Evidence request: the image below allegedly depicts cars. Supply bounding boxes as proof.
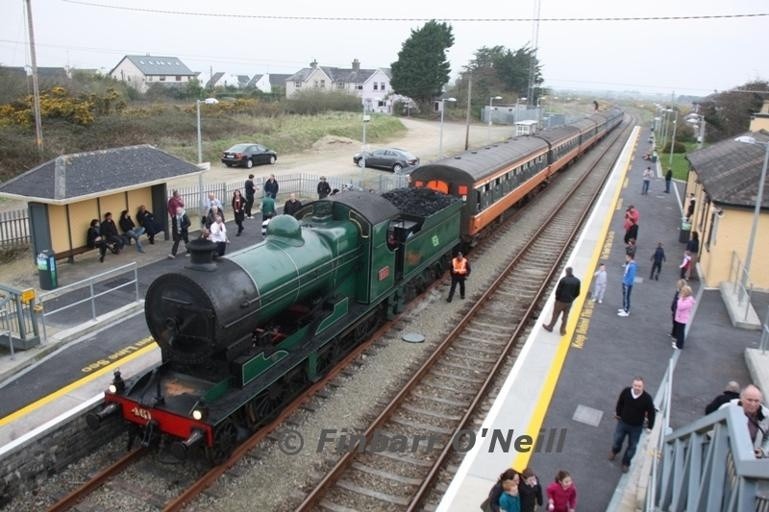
[353,146,420,173]
[221,143,277,168]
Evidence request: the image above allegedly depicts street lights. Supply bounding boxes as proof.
[537,95,583,127]
[516,97,528,120]
[196,97,220,215]
[489,95,502,143]
[652,100,679,166]
[690,113,706,151]
[362,96,383,144]
[734,135,769,305]
[439,97,457,156]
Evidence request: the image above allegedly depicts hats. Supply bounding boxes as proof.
[320,176,326,180]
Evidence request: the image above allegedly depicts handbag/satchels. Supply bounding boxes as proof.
[481,496,496,512]
[112,244,120,254]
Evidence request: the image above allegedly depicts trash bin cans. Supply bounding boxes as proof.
[679,223,691,242]
[36,250,58,290]
[652,156,657,163]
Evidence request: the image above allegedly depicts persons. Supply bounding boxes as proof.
[617,252,638,318]
[447,251,471,300]
[546,470,577,512]
[720,384,768,458]
[87,219,118,263]
[168,173,340,260]
[642,167,654,194]
[672,285,696,350]
[101,212,128,254]
[499,478,521,511]
[137,205,161,244]
[621,192,701,283]
[610,375,655,472]
[592,263,608,303]
[667,279,687,338]
[663,167,672,193]
[542,267,580,335]
[119,210,146,253]
[704,381,740,439]
[521,467,543,511]
[487,469,522,511]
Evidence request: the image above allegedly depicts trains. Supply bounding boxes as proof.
[92,105,625,475]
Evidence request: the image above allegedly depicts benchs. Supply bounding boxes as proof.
[54,234,131,264]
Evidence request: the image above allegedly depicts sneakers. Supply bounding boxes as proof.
[617,309,628,316]
[608,450,615,460]
[543,323,552,332]
[622,465,628,472]
